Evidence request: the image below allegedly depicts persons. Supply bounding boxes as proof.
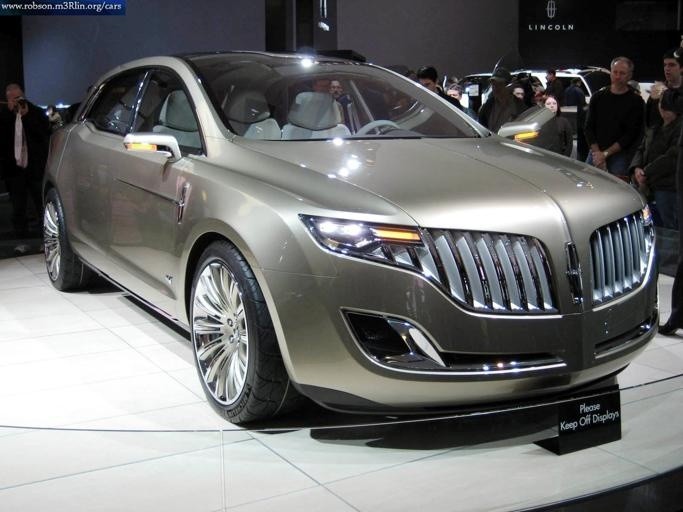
[655,245,681,336]
[581,57,645,176]
[46,104,61,126]
[310,54,587,147]
[625,93,681,230]
[62,86,91,124]
[0,83,54,254]
[644,52,681,132]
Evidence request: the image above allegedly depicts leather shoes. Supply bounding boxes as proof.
[658,322,683,337]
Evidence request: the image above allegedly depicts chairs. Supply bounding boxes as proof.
[224,85,281,140]
[153,91,202,150]
[281,91,352,140]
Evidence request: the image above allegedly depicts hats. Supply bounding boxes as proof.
[488,67,513,82]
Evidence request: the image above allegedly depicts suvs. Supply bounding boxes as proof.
[40,50,666,424]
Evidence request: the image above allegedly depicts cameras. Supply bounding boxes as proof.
[13,96,25,108]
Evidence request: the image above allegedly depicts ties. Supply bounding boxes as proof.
[13,113,24,162]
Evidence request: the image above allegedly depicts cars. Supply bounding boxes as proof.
[39,101,76,125]
[436,65,671,164]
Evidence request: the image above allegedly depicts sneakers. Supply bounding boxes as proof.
[14,243,45,254]
